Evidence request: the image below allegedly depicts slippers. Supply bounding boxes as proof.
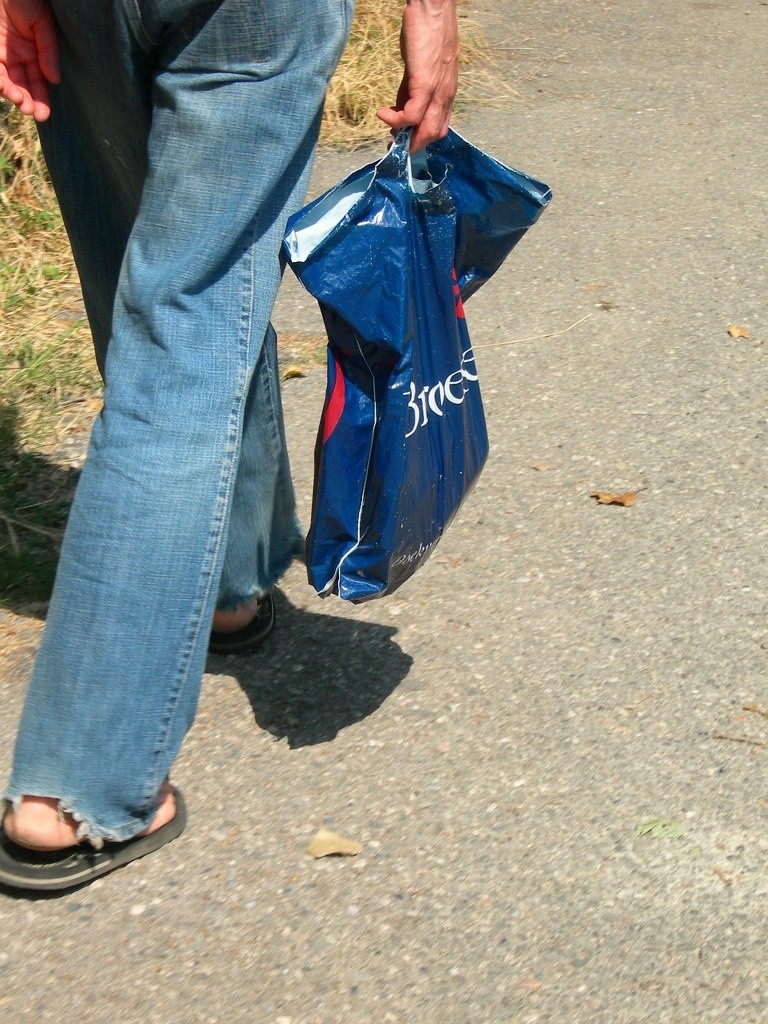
[206,589,272,654]
[0,784,186,890]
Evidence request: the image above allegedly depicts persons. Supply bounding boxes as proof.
[0,0,459,890]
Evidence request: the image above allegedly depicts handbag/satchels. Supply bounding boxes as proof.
[284,124,552,603]
